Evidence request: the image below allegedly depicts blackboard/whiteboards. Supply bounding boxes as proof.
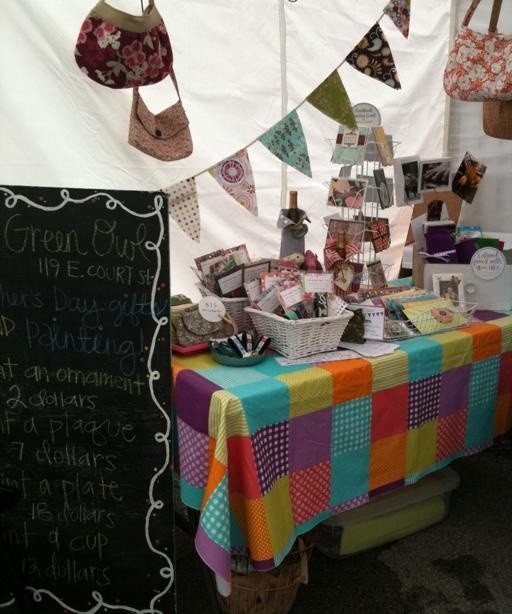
[0,184,179,614]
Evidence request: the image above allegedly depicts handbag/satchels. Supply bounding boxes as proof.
[171,303,222,347]
[75,0,193,86]
[128,86,193,161]
[443,0,512,139]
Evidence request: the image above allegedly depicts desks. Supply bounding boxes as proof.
[161,302,511,614]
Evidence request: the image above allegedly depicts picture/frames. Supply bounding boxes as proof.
[432,272,465,310]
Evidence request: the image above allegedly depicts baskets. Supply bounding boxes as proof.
[196,281,355,359]
[216,533,318,614]
[383,299,479,341]
